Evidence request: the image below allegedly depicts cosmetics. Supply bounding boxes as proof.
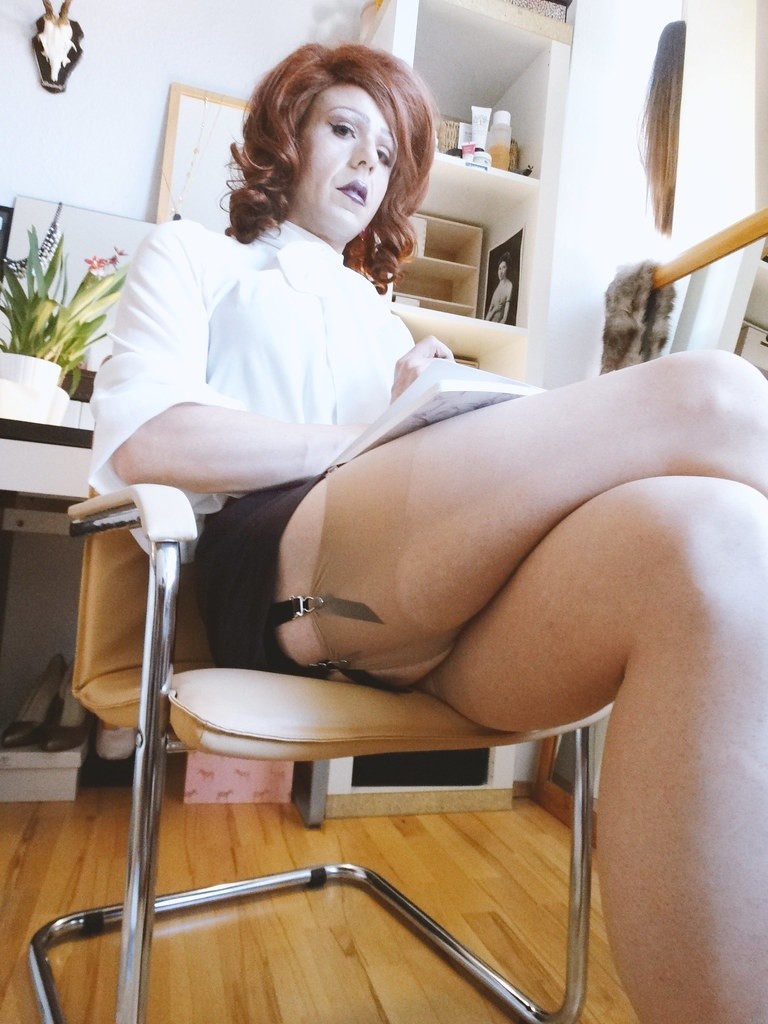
[446,104,512,169]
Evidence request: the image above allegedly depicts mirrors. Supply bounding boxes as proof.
[155,82,251,240]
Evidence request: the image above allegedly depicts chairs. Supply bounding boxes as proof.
[22,485,617,1024]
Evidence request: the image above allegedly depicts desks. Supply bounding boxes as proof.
[0,416,95,803]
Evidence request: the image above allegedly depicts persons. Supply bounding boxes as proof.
[484,254,511,324]
[88,46,768,1024]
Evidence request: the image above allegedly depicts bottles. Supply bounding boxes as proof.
[488,110,512,169]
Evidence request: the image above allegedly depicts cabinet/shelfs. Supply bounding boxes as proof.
[321,0,577,826]
[393,212,483,316]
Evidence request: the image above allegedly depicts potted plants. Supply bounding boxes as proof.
[0,225,131,423]
